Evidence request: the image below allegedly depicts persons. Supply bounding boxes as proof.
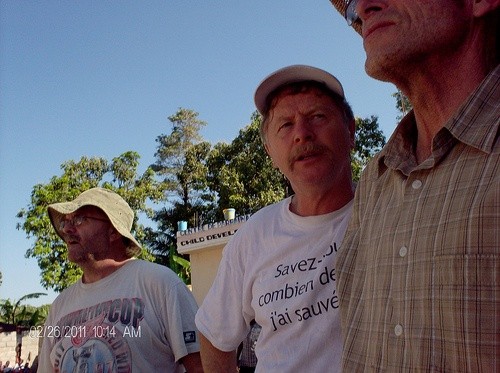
[0,355,38,373]
[329,0,500,372]
[195,64,359,373]
[35,188,205,373]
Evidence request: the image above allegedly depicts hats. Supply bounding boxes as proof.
[46,187,143,258]
[253,65,346,115]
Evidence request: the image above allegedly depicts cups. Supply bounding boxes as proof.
[177,221,187,230]
[222,208,235,220]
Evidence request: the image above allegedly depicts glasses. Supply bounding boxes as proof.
[345,0,363,26]
[58,214,110,231]
